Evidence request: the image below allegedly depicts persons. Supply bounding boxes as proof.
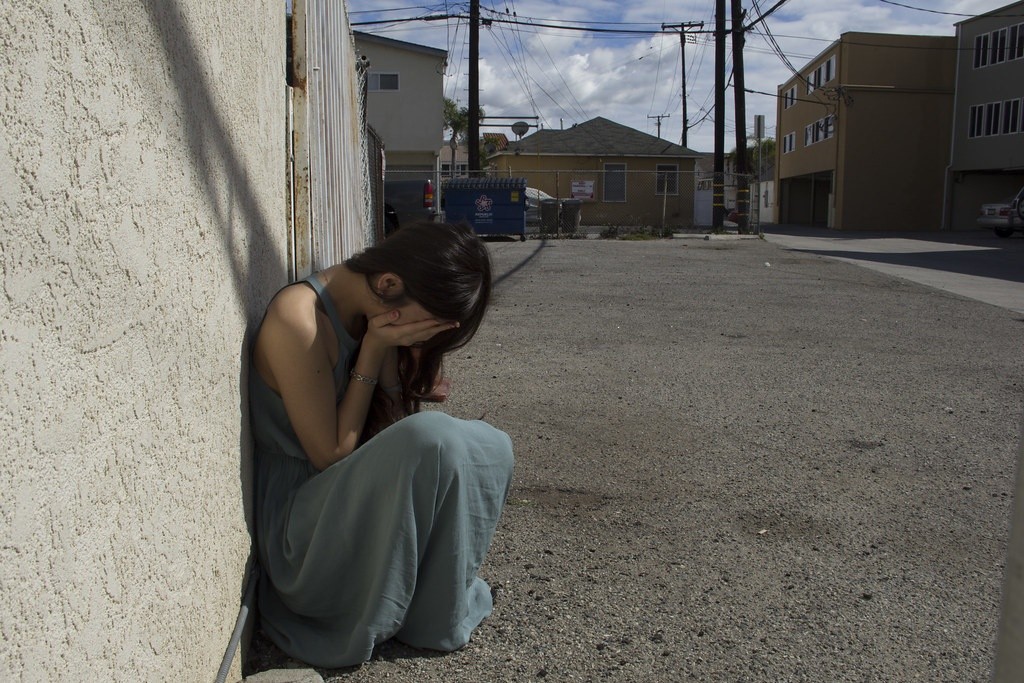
[247,220,514,669]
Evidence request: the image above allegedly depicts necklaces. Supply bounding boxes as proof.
[320,270,329,290]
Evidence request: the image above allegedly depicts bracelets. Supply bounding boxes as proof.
[350,367,377,386]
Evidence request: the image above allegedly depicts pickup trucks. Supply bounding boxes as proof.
[976,184,1024,239]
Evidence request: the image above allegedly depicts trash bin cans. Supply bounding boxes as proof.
[539,198,560,235]
[561,197,584,234]
[440,176,530,242]
